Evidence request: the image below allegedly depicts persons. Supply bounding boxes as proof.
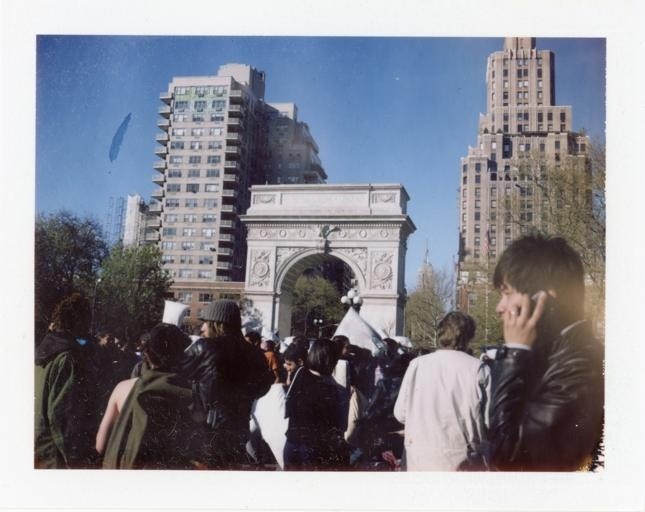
[35,292,496,471]
[473,231,606,471]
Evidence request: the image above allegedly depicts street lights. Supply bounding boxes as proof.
[478,277,489,344]
[89,277,103,337]
[343,288,363,314]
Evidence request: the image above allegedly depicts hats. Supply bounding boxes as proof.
[197,299,241,325]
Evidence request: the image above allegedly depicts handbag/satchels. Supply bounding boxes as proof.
[284,396,291,418]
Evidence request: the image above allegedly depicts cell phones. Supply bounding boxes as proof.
[529,292,558,329]
